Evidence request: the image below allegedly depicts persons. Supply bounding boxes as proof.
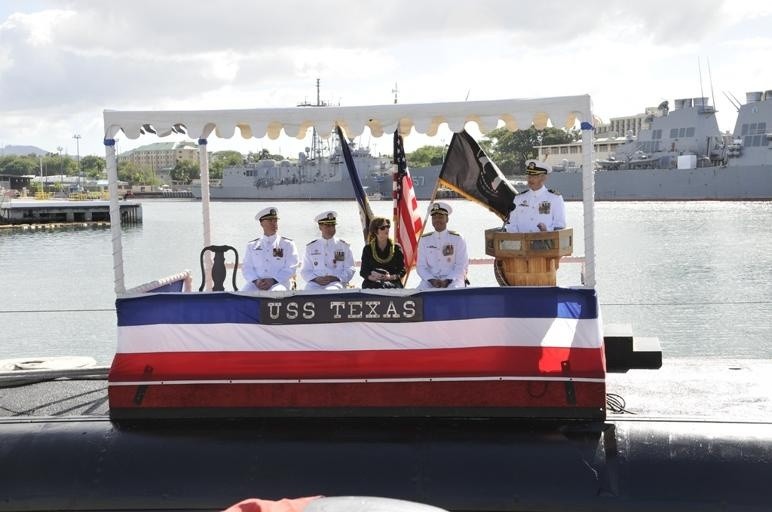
[300,210,356,290]
[359,218,406,288]
[504,158,566,232]
[234,206,302,293]
[415,201,468,288]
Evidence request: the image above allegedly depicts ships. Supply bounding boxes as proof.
[189,78,442,199]
[507,90,772,200]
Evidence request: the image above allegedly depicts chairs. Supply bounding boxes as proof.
[198,243,240,292]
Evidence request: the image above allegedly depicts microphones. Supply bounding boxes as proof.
[503,202,517,220]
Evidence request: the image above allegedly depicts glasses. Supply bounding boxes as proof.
[377,224,390,230]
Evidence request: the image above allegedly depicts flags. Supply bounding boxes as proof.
[441,127,519,223]
[335,126,375,243]
[392,126,422,268]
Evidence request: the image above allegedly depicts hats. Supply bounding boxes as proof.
[524,159,552,175]
[427,201,452,216]
[255,206,278,220]
[314,211,338,224]
